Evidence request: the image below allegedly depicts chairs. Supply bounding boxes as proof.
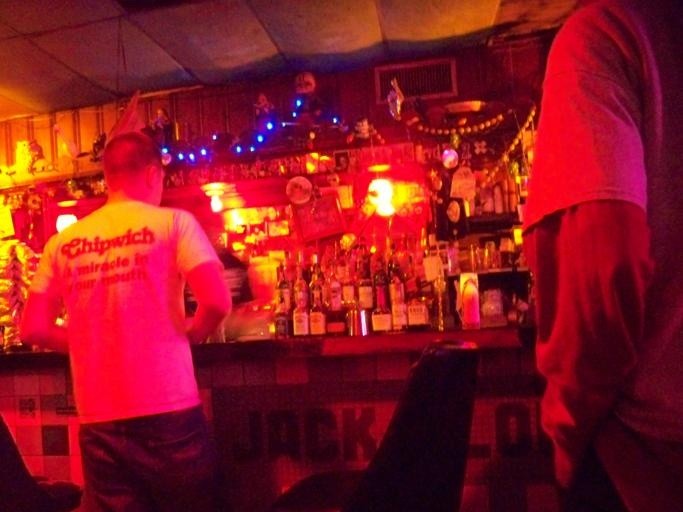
[273,344,480,512]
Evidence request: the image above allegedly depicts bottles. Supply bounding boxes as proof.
[59,136,78,176]
[276,145,525,337]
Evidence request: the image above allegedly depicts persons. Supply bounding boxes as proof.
[14,130,232,512]
[519,0,680,510]
[159,198,274,344]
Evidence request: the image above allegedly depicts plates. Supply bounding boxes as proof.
[184,267,245,305]
